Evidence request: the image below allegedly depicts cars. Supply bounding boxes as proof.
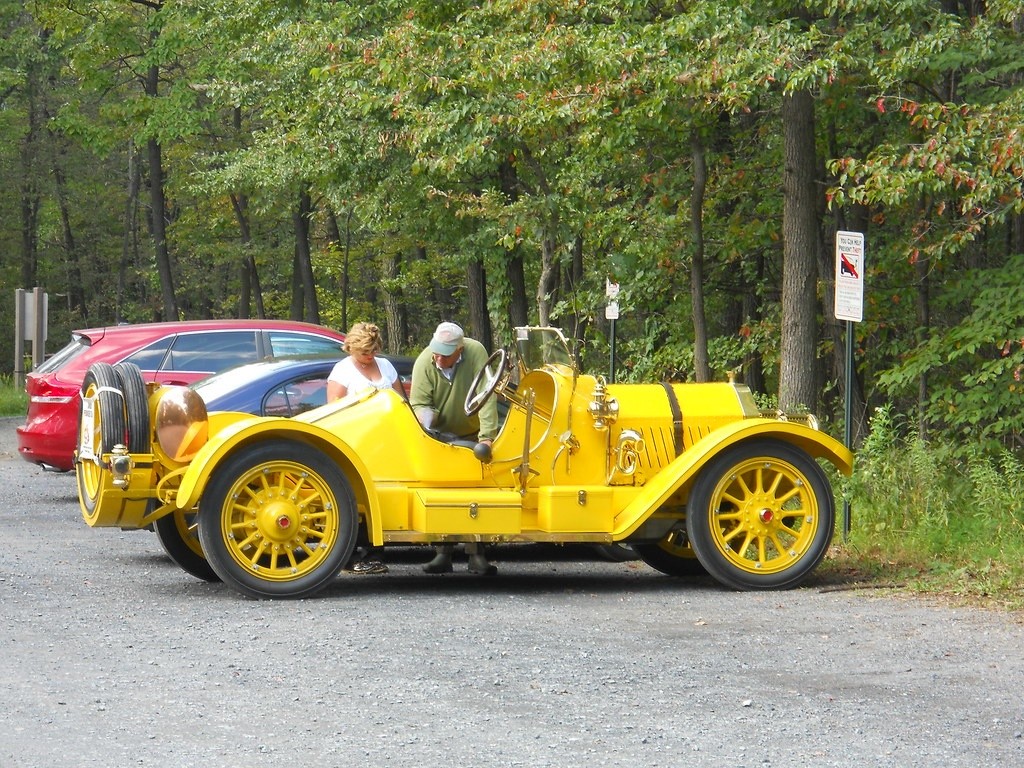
[70,322,857,602]
[119,351,520,453]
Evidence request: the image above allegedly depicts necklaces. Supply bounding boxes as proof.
[362,364,367,368]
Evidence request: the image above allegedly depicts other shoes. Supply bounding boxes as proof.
[468,554,497,575]
[421,554,453,573]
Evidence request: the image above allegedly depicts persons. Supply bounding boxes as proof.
[327,322,407,572]
[410,321,498,574]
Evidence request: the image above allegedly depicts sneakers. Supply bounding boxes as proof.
[347,562,372,573]
[367,561,389,572]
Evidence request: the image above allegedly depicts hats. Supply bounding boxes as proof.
[429,322,464,356]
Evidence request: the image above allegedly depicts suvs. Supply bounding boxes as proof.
[14,317,413,476]
